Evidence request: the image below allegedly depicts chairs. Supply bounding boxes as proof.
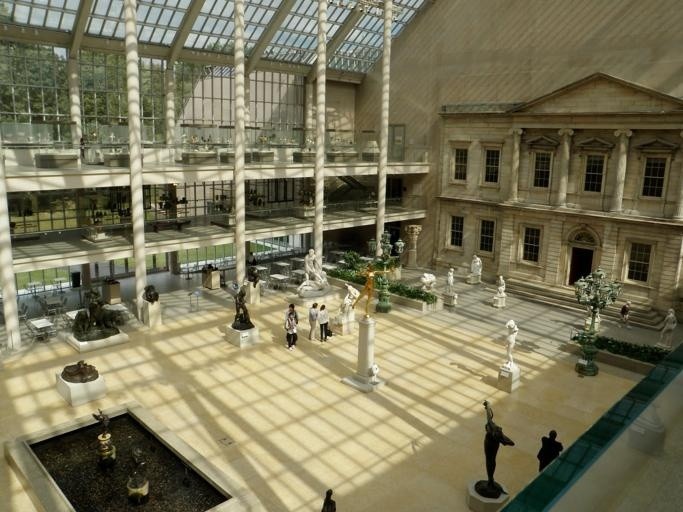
[17,290,68,339]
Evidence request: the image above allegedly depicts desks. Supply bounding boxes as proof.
[252,254,338,287]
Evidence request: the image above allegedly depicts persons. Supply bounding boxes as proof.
[615,301,633,329]
[284,303,299,351]
[658,307,677,346]
[352,263,395,316]
[322,489,336,512]
[505,319,519,367]
[234,287,251,323]
[537,429,563,473]
[336,283,360,319]
[297,249,329,296]
[482,398,516,487]
[447,254,508,298]
[308,303,332,342]
